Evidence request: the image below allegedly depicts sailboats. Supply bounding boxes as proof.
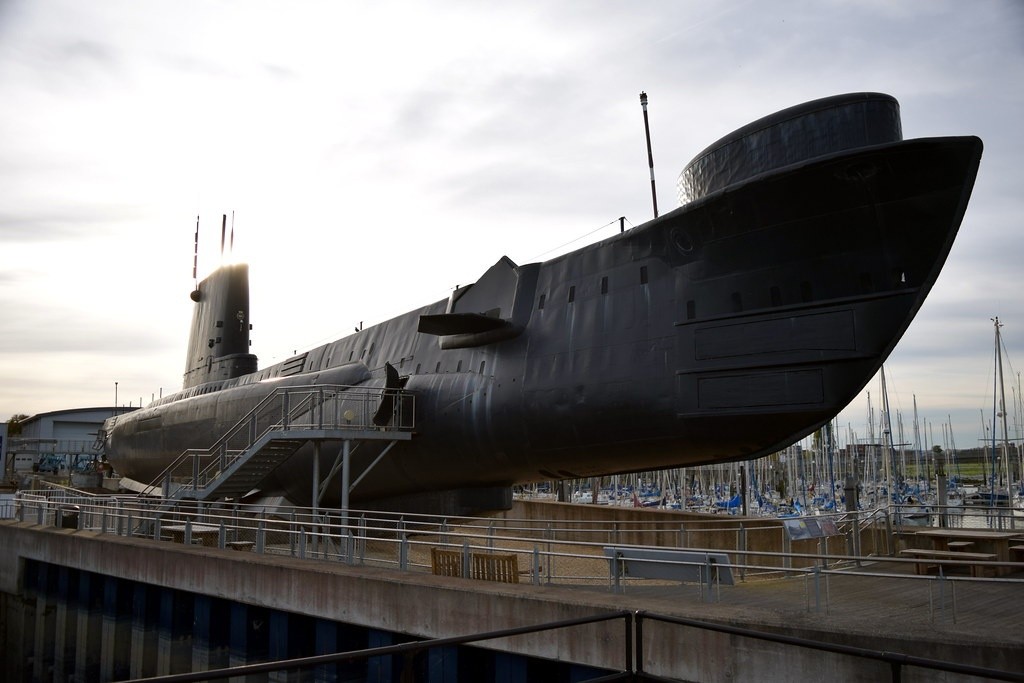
[511,315,1024,531]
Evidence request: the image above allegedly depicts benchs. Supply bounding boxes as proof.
[431,546,542,583]
[900,539,1024,579]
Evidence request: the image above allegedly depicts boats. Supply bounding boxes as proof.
[92,88,986,540]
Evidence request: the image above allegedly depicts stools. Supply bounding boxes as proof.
[229,541,256,551]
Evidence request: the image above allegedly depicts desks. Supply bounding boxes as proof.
[914,529,1024,578]
[161,525,235,547]
